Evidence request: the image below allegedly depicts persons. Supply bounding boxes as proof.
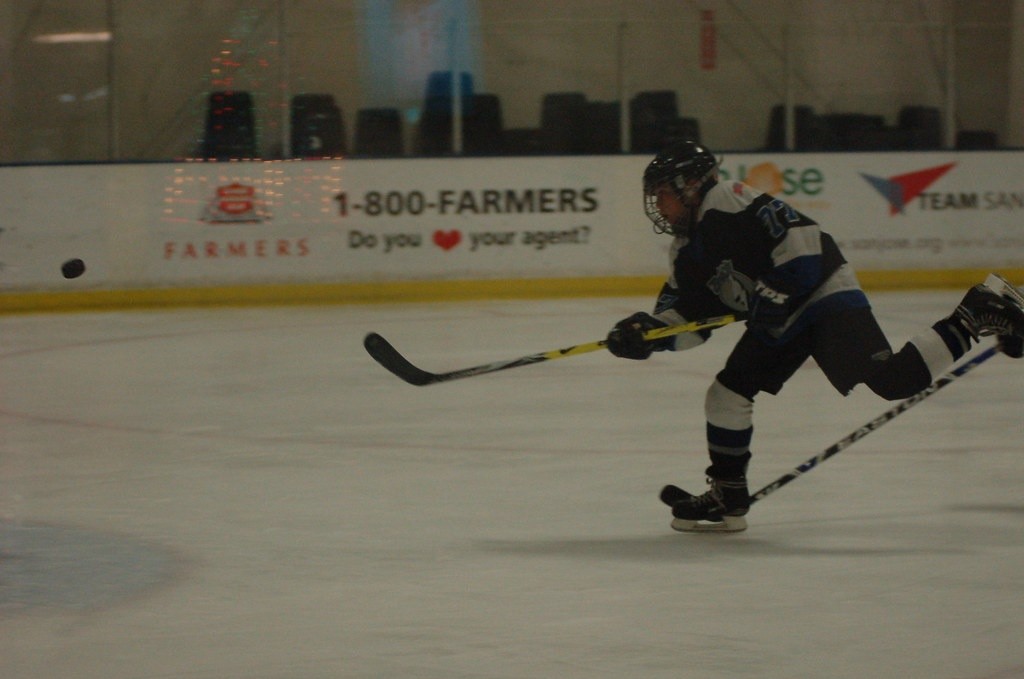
[606,140,1024,521]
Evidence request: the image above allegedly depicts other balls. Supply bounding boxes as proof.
[61,258,85,278]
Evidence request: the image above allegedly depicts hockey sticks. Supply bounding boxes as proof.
[362,305,752,389]
[656,344,1003,513]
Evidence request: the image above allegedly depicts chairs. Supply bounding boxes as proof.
[204,71,705,157]
[766,103,999,151]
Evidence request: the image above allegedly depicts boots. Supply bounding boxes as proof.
[671,451,752,533]
[949,272,1024,358]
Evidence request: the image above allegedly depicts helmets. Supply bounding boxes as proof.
[643,139,724,238]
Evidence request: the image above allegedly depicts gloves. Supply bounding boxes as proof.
[748,282,790,332]
[607,312,676,360]
